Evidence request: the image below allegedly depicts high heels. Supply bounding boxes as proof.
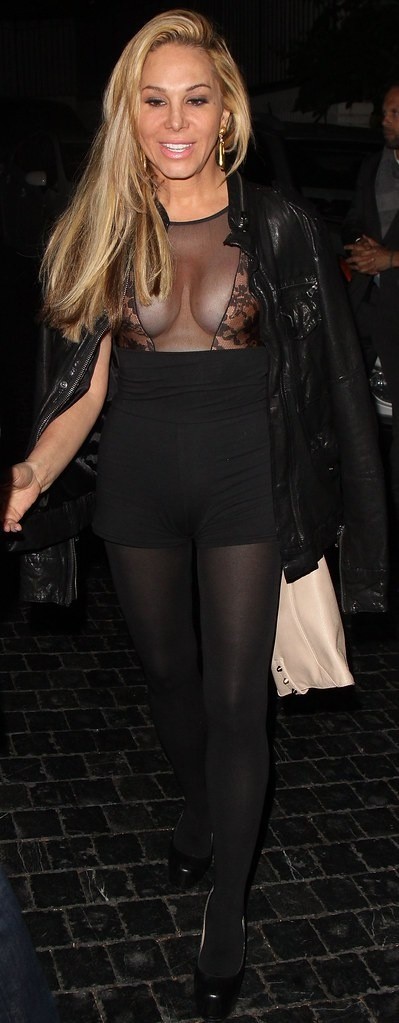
[194,884,247,1023]
[167,807,214,891]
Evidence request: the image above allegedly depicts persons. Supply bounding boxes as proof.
[3,8,339,1022]
[337,83,399,641]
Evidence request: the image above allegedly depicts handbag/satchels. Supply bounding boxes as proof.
[271,548,357,697]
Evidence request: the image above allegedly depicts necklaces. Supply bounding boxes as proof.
[394,149,399,164]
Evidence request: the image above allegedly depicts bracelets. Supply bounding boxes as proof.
[387,250,395,267]
